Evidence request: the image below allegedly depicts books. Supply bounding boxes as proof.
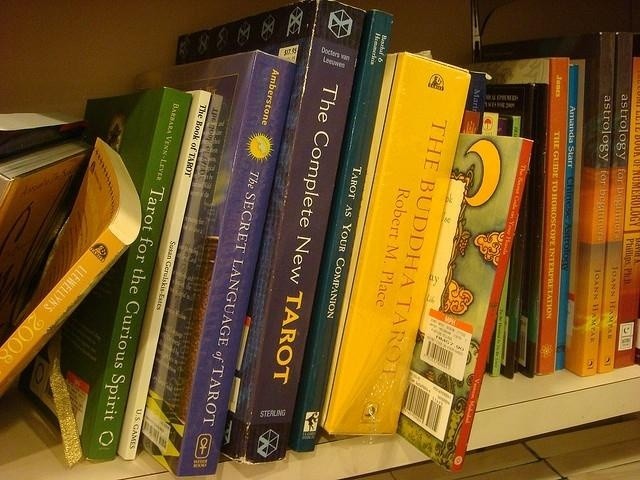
[0,87,211,470]
[135,1,640,479]
